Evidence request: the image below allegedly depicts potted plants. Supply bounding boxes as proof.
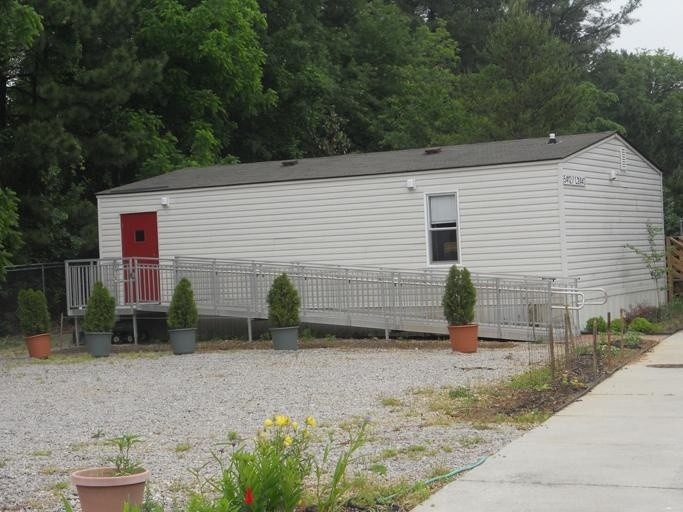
[265,271,302,350]
[441,264,478,353]
[71,433,149,512]
[166,277,198,354]
[15,288,51,358]
[81,281,117,357]
